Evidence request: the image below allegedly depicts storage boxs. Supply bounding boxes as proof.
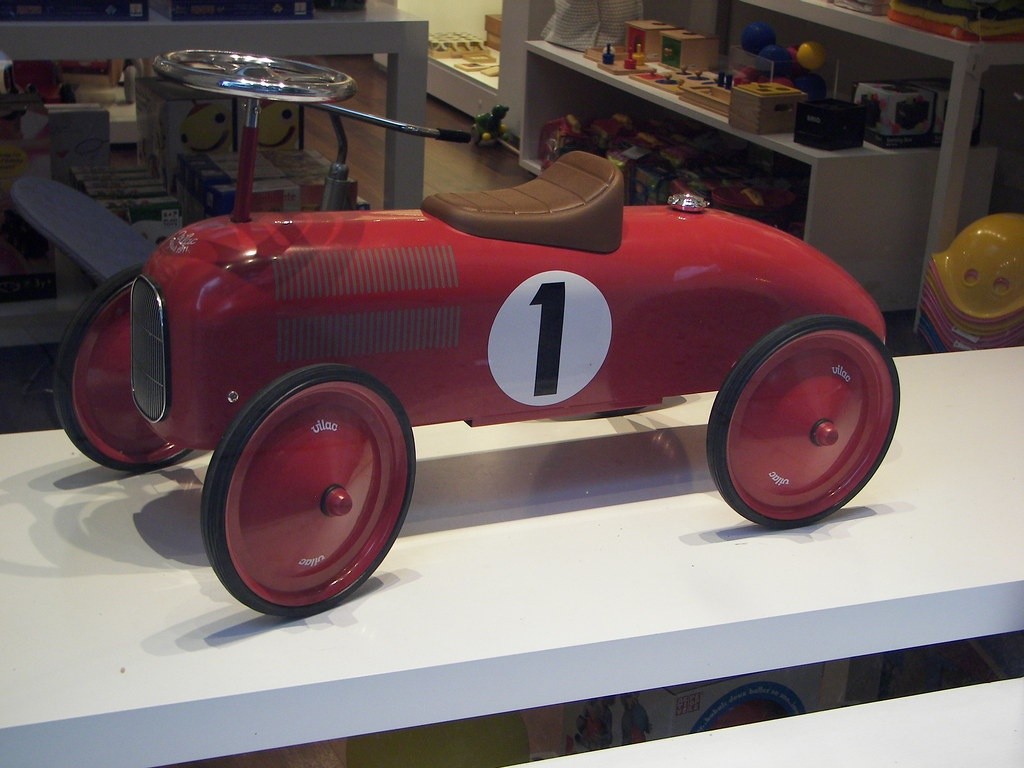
[728,82,808,135]
[794,97,866,151]
[561,661,824,755]
[906,78,984,148]
[854,81,938,147]
[624,19,677,63]
[658,28,721,73]
[485,14,502,51]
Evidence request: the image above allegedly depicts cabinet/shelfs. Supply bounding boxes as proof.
[516,0,1024,319]
[0,0,433,388]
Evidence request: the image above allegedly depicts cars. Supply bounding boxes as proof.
[53,48,903,624]
